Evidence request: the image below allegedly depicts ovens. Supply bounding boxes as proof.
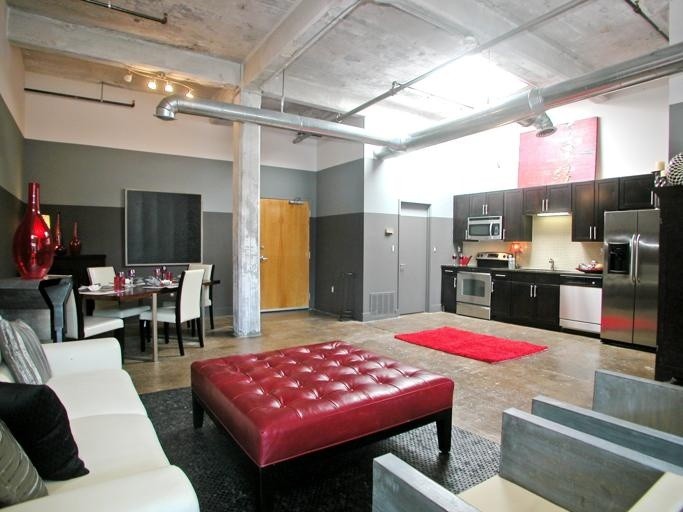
[455,272,491,307]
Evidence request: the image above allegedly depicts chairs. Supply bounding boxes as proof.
[532,365,683,469]
[0,254,222,359]
[369,405,683,512]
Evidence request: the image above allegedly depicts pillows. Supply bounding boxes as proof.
[0,316,54,386]
[0,420,48,505]
[1,382,88,483]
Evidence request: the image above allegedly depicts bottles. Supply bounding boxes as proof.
[52,212,66,255]
[453,254,457,265]
[458,253,463,265]
[68,221,81,255]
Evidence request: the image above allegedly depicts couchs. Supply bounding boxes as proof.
[0,312,200,512]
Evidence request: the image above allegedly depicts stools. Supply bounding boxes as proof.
[191,339,454,509]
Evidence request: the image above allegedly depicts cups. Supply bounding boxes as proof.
[154,265,173,282]
[113,271,126,292]
[128,269,136,277]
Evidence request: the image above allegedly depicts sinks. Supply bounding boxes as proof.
[523,268,566,273]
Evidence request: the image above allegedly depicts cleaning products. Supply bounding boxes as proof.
[508,255,515,270]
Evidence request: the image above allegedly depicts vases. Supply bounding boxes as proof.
[69,221,82,255]
[48,209,66,254]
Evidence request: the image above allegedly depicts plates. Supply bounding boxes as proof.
[576,266,602,273]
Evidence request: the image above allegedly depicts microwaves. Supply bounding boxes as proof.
[467,217,502,240]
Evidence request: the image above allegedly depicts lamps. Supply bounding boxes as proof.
[121,68,193,100]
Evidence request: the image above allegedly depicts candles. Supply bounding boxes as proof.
[655,159,665,170]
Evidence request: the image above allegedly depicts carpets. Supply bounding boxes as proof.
[394,324,551,366]
[136,385,501,511]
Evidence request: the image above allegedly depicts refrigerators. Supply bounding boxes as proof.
[599,209,659,348]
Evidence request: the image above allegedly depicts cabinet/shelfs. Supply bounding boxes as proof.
[513,271,559,330]
[572,177,618,244]
[438,263,455,311]
[490,270,514,323]
[501,188,533,242]
[452,194,470,234]
[619,173,660,211]
[523,185,572,217]
[652,184,682,390]
[471,189,502,218]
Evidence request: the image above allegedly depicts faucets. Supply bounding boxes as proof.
[548,258,555,270]
